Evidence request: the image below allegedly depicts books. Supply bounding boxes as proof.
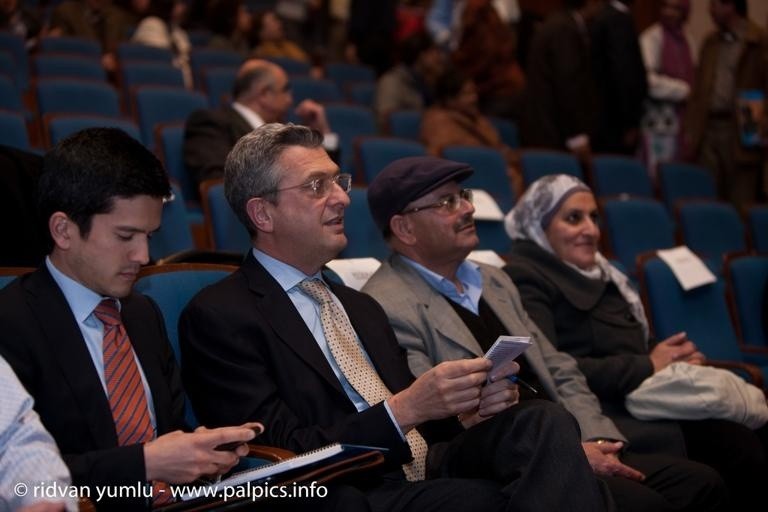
[181,440,344,504]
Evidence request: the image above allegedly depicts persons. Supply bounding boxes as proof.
[501,172,767,510]
[177,122,611,511]
[359,156,730,512]
[0,0,767,267]
[1,356,79,511]
[1,126,371,511]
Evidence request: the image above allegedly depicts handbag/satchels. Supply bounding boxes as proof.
[625,364,767,433]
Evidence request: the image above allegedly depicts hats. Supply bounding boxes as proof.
[367,153,473,232]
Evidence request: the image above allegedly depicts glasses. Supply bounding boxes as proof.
[260,170,357,200]
[406,186,477,214]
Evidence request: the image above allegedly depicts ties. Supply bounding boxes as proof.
[92,298,180,505]
[299,275,430,486]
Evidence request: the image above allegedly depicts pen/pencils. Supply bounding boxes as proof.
[507,375,537,394]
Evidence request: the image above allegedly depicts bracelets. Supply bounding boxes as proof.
[594,438,607,445]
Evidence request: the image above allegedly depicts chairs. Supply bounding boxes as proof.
[0,34,768,512]
